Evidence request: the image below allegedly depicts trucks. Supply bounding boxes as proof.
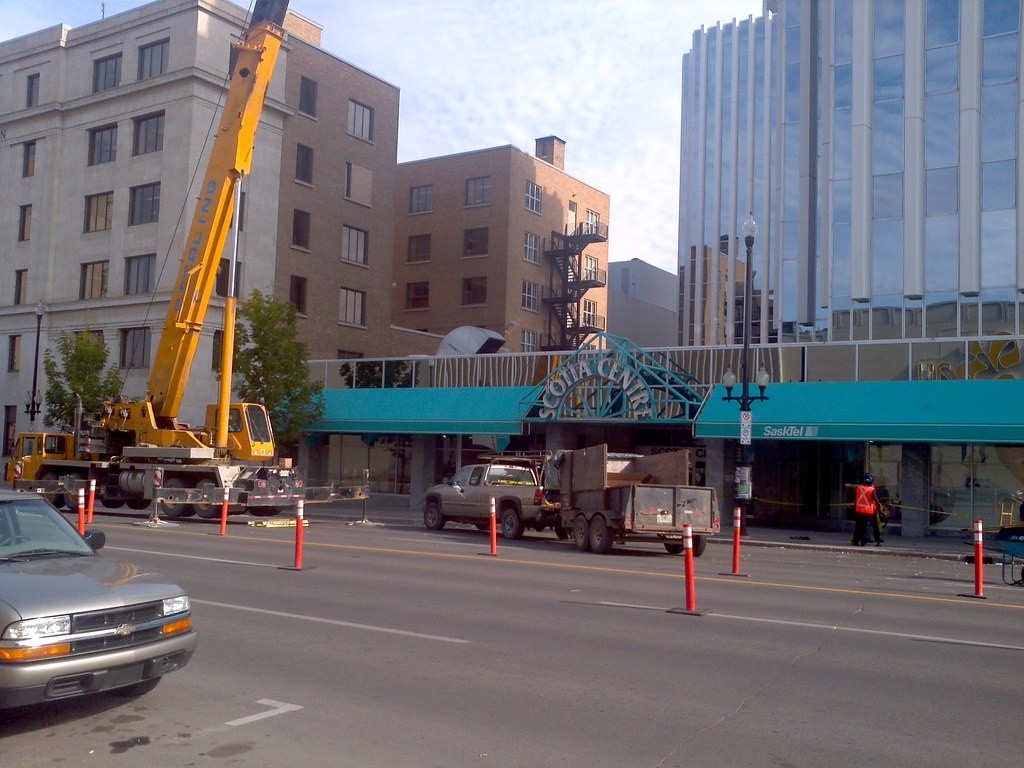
[546,443,720,556]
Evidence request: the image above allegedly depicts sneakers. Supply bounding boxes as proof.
[851,540,860,546]
[876,544,882,547]
[866,539,874,542]
[875,538,884,542]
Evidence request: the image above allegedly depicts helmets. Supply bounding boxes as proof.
[862,472,874,483]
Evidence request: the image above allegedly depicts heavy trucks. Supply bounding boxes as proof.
[5,430,305,517]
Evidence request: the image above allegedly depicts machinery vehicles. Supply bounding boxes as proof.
[76,0,296,473]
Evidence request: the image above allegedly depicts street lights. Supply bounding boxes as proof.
[721,209,771,537]
[22,299,42,454]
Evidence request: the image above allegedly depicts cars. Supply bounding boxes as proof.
[1,487,198,716]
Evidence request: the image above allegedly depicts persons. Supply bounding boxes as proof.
[848,472,885,548]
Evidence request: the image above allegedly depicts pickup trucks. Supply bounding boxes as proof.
[423,449,573,541]
